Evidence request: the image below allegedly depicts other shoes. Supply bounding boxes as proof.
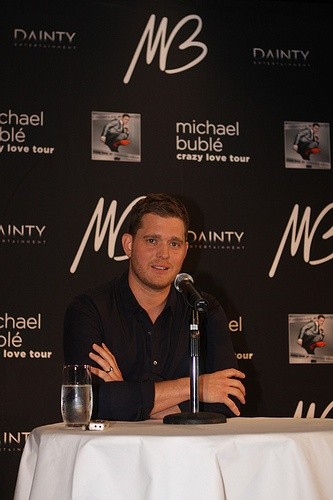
[113,139,130,148]
[309,341,326,350]
[305,148,320,156]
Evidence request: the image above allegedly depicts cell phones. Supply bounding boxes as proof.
[89,419,109,431]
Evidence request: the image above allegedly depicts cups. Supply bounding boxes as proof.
[58,365,94,430]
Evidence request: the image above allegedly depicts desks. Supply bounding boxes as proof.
[12,417,332,499]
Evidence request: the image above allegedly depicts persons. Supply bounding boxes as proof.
[297,315,327,363]
[292,123,321,168]
[100,114,130,161]
[62,192,247,422]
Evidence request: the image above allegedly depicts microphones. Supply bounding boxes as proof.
[174,273,209,313]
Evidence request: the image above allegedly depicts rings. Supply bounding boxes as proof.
[104,367,113,373]
